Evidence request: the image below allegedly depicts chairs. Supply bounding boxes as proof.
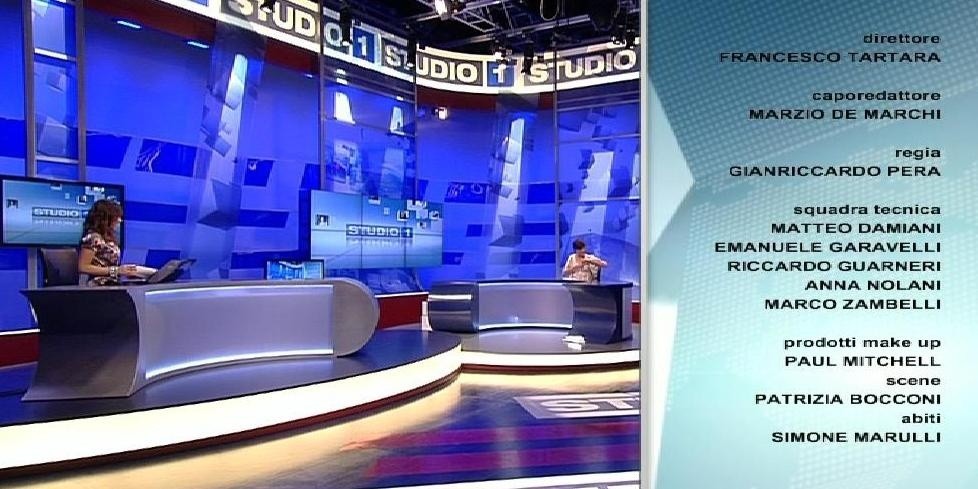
[42,248,79,285]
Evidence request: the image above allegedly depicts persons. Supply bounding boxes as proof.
[76,200,137,286]
[562,240,607,283]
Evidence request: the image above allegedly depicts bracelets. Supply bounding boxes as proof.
[109,265,119,278]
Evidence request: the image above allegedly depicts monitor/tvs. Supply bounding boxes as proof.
[264,259,324,280]
[0,175,125,247]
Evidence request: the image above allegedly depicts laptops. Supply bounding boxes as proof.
[122,260,182,284]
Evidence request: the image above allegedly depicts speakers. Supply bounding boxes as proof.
[585,1,621,32]
